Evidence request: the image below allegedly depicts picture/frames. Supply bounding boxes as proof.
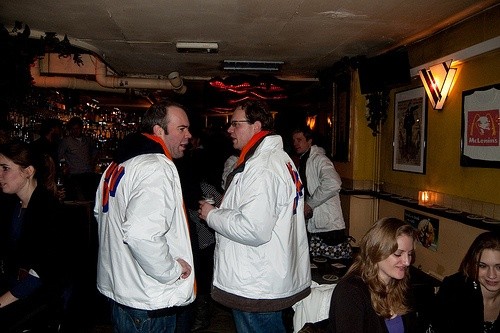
[331,69,353,162]
[459,83,500,170]
[391,86,428,175]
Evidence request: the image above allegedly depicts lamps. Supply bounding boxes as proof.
[418,59,457,110]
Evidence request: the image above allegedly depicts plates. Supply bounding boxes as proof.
[466,215,485,219]
[313,258,327,263]
[483,219,500,223]
[323,274,338,282]
[391,196,403,198]
[383,194,392,195]
[446,211,462,213]
[432,207,448,209]
[408,200,418,203]
[399,198,411,200]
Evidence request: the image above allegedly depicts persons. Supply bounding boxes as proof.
[0,139,82,333]
[58,117,92,188]
[291,125,346,246]
[198,99,312,333]
[34,154,66,199]
[93,100,197,333]
[432,232,500,333]
[329,217,432,333]
[191,132,203,149]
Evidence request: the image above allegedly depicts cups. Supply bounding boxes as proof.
[202,194,216,207]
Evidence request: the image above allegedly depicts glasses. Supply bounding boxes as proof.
[231,120,248,128]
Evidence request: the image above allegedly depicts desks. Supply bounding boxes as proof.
[292,255,351,333]
